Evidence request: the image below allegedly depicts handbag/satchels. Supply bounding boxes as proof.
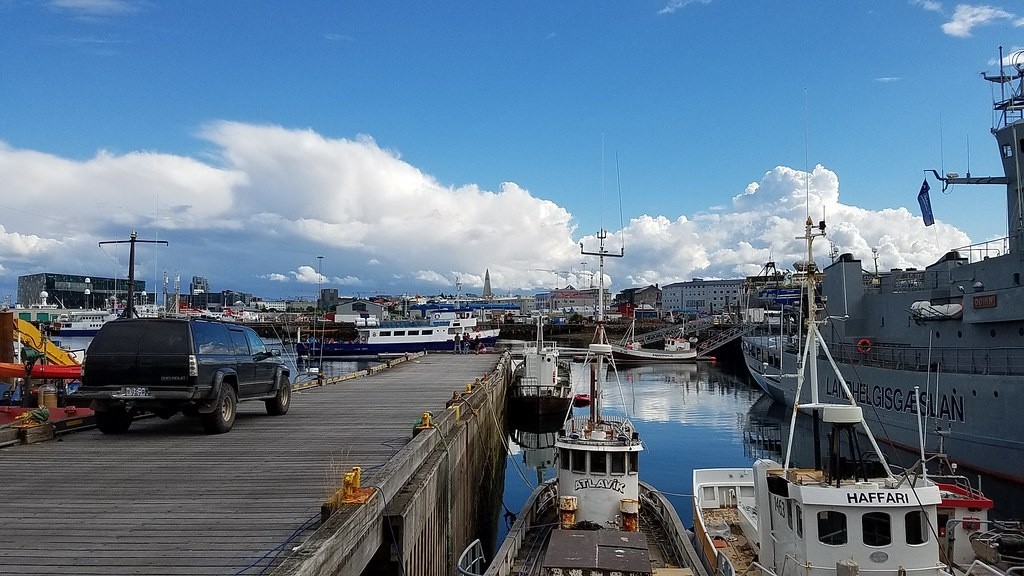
[482,347,487,353]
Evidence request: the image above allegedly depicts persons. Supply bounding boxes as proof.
[306,335,334,344]
[453,332,483,355]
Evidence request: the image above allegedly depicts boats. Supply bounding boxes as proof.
[606,309,699,361]
[49,308,119,338]
[295,316,501,359]
[692,217,1024,576]
[455,227,735,576]
[504,308,574,412]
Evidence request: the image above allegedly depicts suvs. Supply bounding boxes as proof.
[70,316,292,436]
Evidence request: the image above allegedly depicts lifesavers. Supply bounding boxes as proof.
[857,339,873,353]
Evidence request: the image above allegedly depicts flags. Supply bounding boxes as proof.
[918,179,934,226]
